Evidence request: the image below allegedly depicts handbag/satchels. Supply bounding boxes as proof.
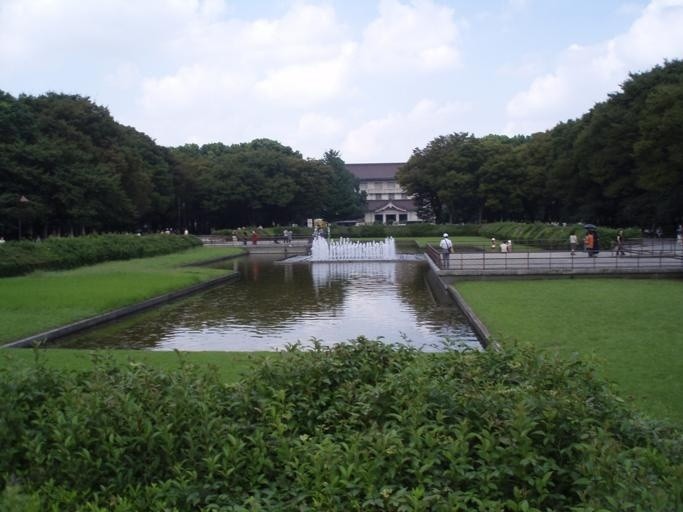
[448,247,452,253]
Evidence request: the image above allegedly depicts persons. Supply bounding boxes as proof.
[676,224,682,246]
[615,230,625,256]
[656,226,664,239]
[439,232,453,269]
[584,230,600,257]
[185,226,189,235]
[231,224,264,246]
[283,222,298,244]
[160,227,174,234]
[569,231,579,256]
[545,220,568,228]
[314,222,330,238]
[490,236,513,253]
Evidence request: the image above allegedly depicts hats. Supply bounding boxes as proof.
[441,233,449,238]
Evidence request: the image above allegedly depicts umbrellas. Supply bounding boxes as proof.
[584,223,596,228]
